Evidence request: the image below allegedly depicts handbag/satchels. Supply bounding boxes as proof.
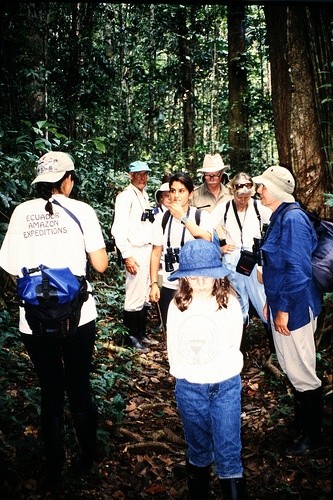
[16,264,90,342]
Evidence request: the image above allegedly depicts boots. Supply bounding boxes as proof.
[123,305,150,349]
[185,458,214,500]
[218,473,248,500]
[286,385,328,458]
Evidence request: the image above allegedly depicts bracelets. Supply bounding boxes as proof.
[182,217,187,224]
[150,281,157,287]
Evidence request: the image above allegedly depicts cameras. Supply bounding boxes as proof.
[141,210,155,223]
[253,238,264,267]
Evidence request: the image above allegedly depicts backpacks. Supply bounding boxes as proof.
[280,207,333,293]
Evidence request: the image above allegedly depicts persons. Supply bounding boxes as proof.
[111,161,156,349]
[0,151,108,471]
[252,166,333,455]
[166,239,244,500]
[148,154,271,327]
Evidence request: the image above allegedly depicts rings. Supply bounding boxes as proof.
[282,329,284,331]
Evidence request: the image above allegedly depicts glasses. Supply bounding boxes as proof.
[184,277,215,285]
[231,178,253,190]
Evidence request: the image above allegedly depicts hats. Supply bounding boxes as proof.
[196,153,230,174]
[167,239,232,280]
[252,166,295,203]
[30,152,75,186]
[155,183,170,206]
[129,161,151,173]
